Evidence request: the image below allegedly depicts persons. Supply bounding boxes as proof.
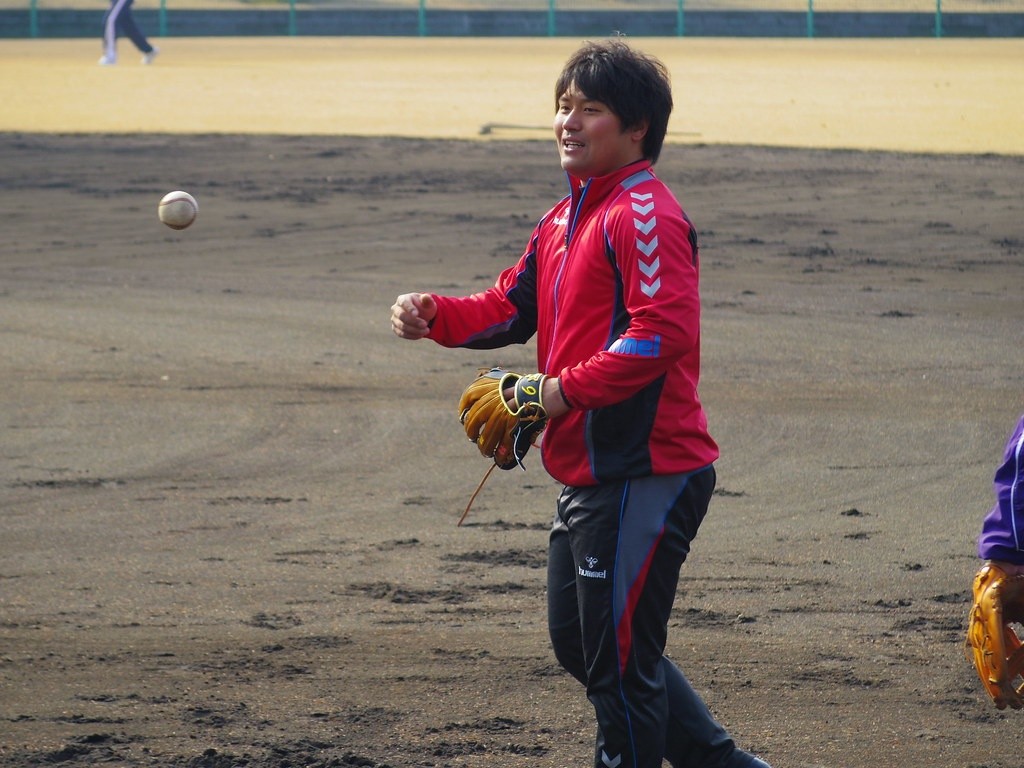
[966,414,1023,710]
[97,0,159,64]
[391,41,772,768]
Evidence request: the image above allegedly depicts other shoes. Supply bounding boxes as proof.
[748,757,772,768]
[97,55,115,64]
[142,47,159,66]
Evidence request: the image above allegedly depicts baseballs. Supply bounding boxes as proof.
[158,190,199,231]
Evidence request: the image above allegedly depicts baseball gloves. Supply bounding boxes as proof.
[457,365,550,473]
[963,563,1024,712]
